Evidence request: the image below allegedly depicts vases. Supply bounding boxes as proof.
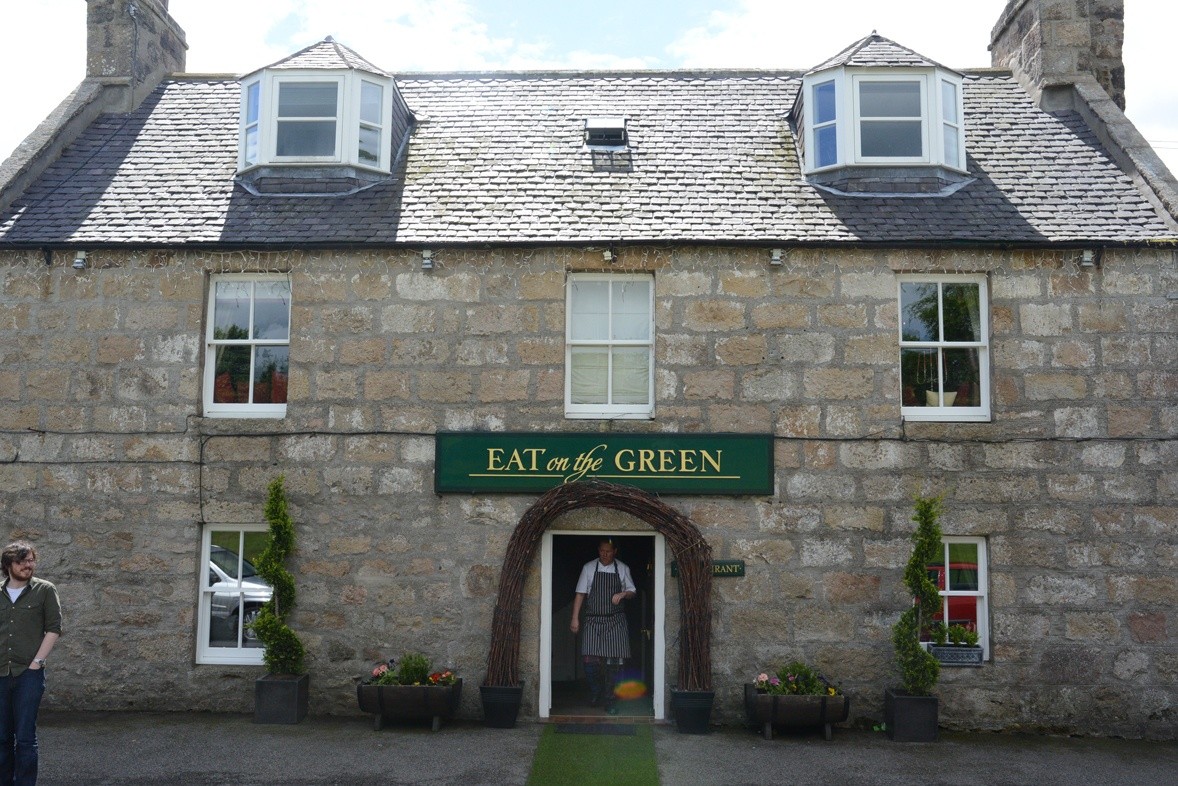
[925,641,983,665]
[745,682,851,742]
[356,679,463,732]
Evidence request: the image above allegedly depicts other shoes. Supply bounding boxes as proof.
[605,704,618,715]
[592,695,601,705]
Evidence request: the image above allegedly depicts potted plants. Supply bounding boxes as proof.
[253,474,310,725]
[881,486,948,742]
[480,476,716,736]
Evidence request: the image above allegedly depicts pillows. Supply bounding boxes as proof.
[216,370,287,401]
[925,390,958,406]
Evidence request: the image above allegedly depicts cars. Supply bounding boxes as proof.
[208,545,277,644]
[917,562,976,630]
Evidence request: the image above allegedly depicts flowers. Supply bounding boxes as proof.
[368,656,459,688]
[752,660,843,697]
[932,618,982,647]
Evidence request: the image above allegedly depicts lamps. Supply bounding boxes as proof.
[769,248,784,266]
[422,249,435,269]
[1081,249,1096,267]
[72,250,90,270]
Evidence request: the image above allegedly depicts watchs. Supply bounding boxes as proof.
[33,658,44,665]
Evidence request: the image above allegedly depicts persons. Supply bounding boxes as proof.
[570,537,636,714]
[0,539,63,786]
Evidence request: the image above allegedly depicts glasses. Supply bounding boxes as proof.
[12,559,36,565]
